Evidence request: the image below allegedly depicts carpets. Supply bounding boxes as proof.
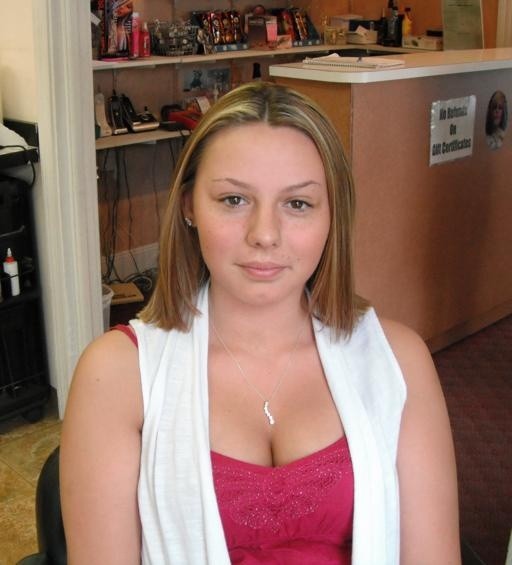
[434,317,512,565]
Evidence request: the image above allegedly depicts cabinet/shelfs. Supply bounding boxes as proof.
[90,0,496,331]
[0,119,59,433]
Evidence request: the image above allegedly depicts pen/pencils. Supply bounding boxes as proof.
[357,56,361,63]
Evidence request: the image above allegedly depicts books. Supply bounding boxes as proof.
[301,53,406,69]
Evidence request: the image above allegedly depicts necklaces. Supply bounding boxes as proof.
[207,312,316,426]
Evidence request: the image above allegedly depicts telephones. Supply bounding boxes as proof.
[169,108,203,130]
[94,85,112,139]
[106,89,129,135]
[120,92,160,133]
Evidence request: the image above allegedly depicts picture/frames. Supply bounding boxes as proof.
[441,0,485,50]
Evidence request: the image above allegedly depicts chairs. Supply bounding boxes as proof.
[16,444,70,565]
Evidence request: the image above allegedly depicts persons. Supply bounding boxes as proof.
[486,91,508,150]
[59,81,464,565]
